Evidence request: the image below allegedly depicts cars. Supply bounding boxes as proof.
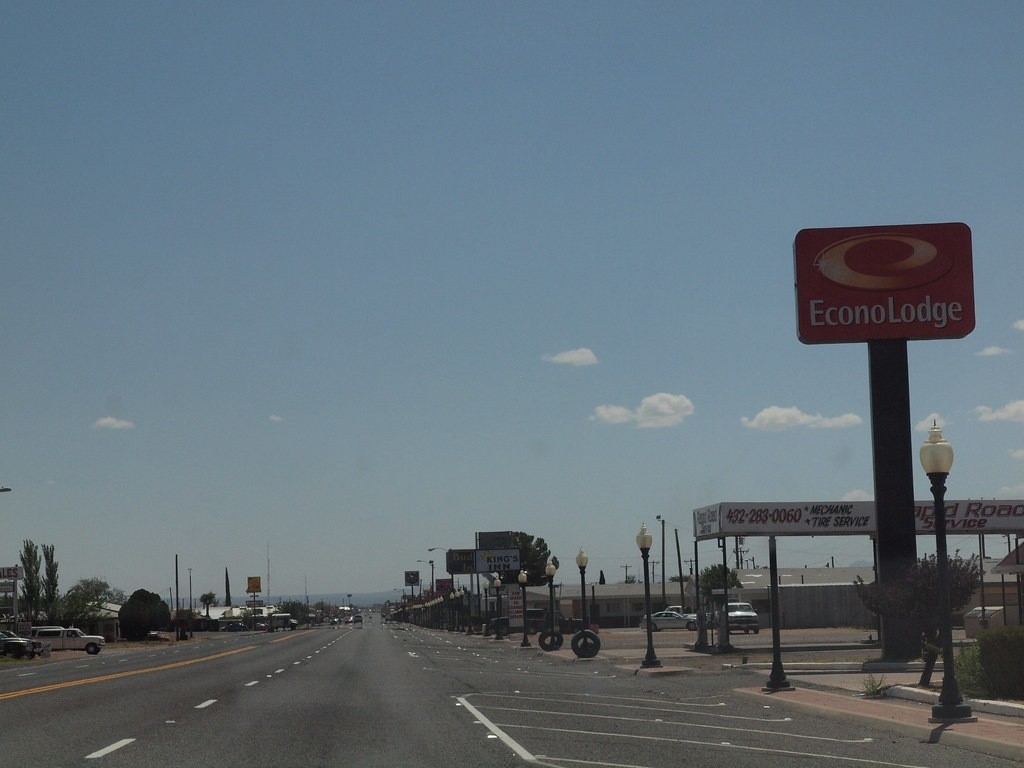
[219,609,392,632]
[715,602,760,634]
[665,605,706,626]
[0,630,32,660]
[1,629,43,660]
[640,610,697,632]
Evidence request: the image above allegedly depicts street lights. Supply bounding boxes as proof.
[518,569,531,647]
[544,558,558,647]
[390,546,475,632]
[482,580,492,637]
[576,546,593,649]
[636,522,664,669]
[918,416,974,720]
[493,573,504,640]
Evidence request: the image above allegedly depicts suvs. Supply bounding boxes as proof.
[33,628,107,655]
